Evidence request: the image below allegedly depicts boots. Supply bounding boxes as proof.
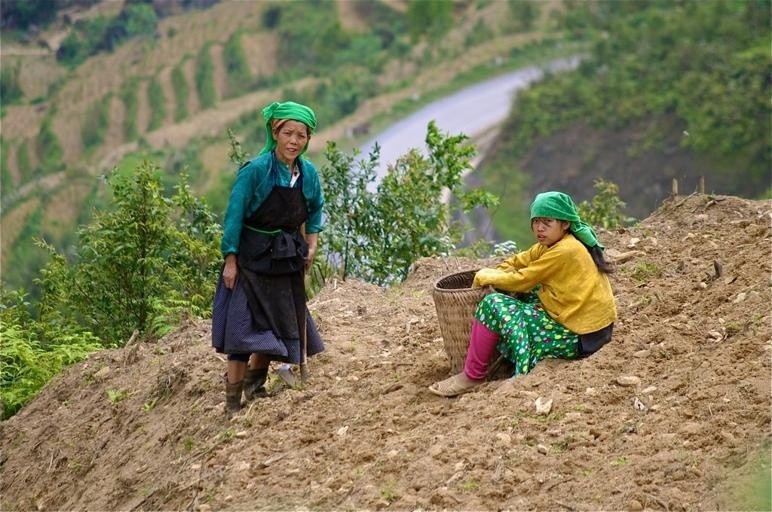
[225,368,271,417]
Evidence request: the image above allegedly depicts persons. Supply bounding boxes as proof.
[429,189,619,399]
[211,99,326,417]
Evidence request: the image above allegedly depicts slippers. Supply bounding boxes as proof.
[428,354,503,397]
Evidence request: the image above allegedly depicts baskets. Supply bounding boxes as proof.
[433,269,520,381]
